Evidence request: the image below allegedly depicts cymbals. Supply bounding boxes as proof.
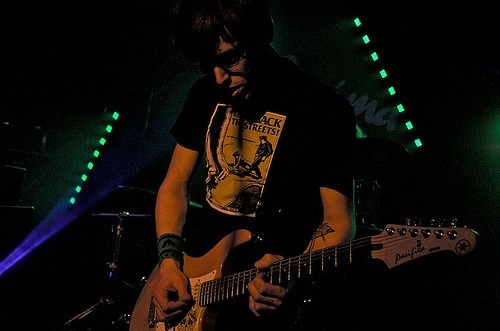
[355,138,402,159]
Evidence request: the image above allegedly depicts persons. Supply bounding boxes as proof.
[151,0,356,331]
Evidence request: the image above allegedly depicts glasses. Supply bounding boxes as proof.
[192,44,247,77]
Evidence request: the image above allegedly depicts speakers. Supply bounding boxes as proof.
[1,165,34,246]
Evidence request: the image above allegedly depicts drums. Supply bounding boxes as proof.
[355,161,387,227]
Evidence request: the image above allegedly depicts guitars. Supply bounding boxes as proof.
[127,216,480,331]
